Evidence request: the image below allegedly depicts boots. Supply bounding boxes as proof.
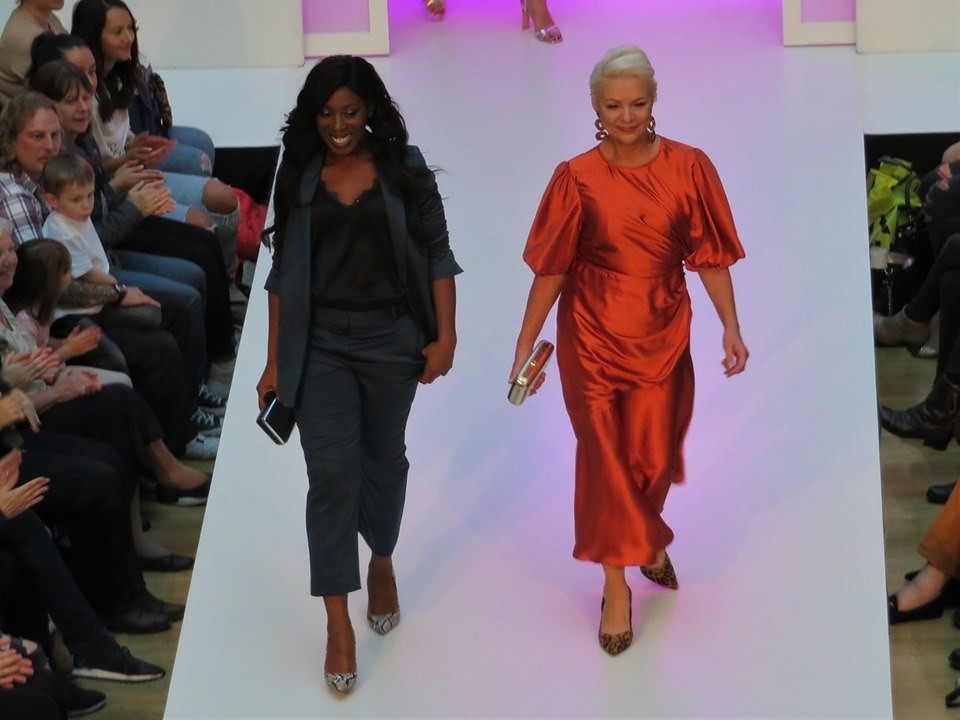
[879,374,960,451]
[873,302,932,358]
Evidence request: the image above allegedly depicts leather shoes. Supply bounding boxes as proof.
[138,589,185,622]
[130,552,195,572]
[106,606,172,635]
[926,482,957,509]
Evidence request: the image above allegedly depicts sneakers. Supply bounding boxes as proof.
[187,407,225,435]
[46,686,107,718]
[181,432,220,459]
[196,383,228,416]
[72,637,166,684]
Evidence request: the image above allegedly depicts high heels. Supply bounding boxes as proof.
[598,585,633,656]
[641,551,680,590]
[424,0,445,20]
[520,1,562,44]
[366,568,401,636]
[323,669,358,694]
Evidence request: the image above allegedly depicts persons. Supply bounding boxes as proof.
[71,0,215,178]
[0,447,167,717]
[420,0,562,44]
[0,217,196,573]
[30,75,237,397]
[22,31,252,315]
[508,42,749,656]
[1,346,185,636]
[255,54,464,695]
[0,0,69,118]
[1,91,236,508]
[0,633,69,720]
[873,134,960,715]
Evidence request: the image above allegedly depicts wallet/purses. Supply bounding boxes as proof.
[255,399,297,445]
[509,340,554,406]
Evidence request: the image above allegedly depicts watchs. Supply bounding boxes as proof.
[112,282,127,308]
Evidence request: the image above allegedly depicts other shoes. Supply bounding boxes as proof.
[889,568,960,708]
[157,472,214,507]
